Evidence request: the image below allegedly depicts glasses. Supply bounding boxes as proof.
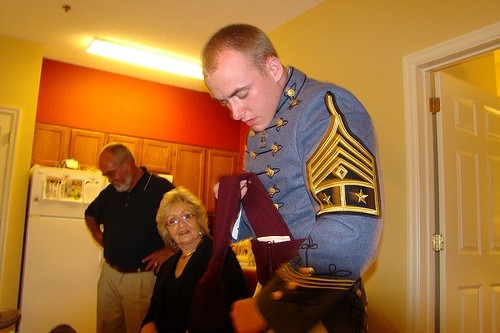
[102,160,125,178]
[166,213,197,226]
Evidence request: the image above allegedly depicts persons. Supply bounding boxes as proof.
[137,186,252,333]
[202,24,382,333]
[84,142,177,333]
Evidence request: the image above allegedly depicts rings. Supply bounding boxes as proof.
[153,261,157,265]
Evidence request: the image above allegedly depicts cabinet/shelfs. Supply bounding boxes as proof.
[33,124,239,216]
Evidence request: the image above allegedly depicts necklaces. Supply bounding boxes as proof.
[180,238,201,259]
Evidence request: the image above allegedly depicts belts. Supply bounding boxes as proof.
[105,259,152,273]
